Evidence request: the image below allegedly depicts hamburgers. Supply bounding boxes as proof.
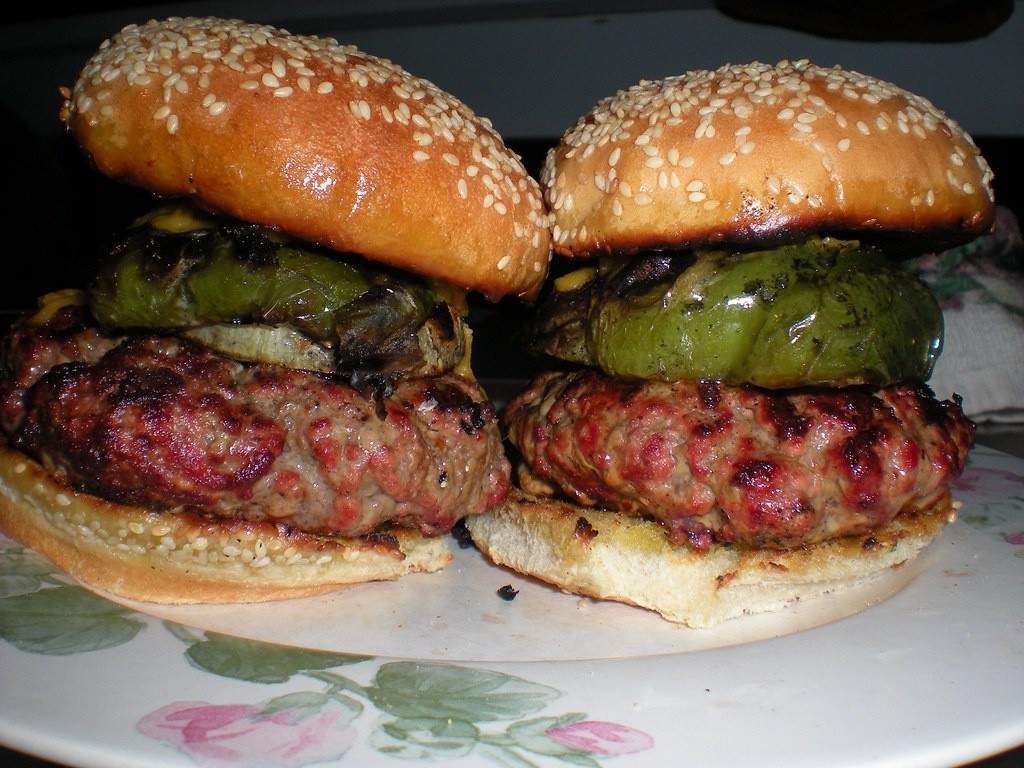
[1,13,554,607]
[458,57,998,628]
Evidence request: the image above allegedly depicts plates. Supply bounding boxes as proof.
[0,444,1024,768]
